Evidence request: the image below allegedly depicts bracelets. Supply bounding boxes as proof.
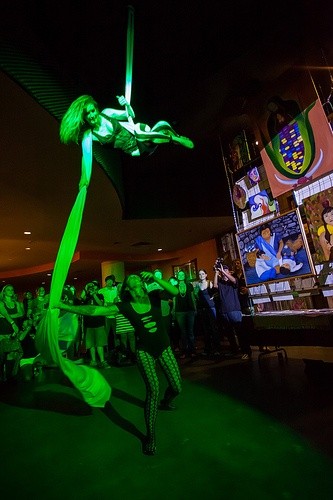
[11,321,15,324]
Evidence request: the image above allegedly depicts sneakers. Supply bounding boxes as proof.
[87,360,110,369]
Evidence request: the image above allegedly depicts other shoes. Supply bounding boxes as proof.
[159,399,176,409]
[143,437,157,455]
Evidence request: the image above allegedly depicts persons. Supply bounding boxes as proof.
[59,95,194,157]
[51,271,184,456]
[0,258,250,390]
